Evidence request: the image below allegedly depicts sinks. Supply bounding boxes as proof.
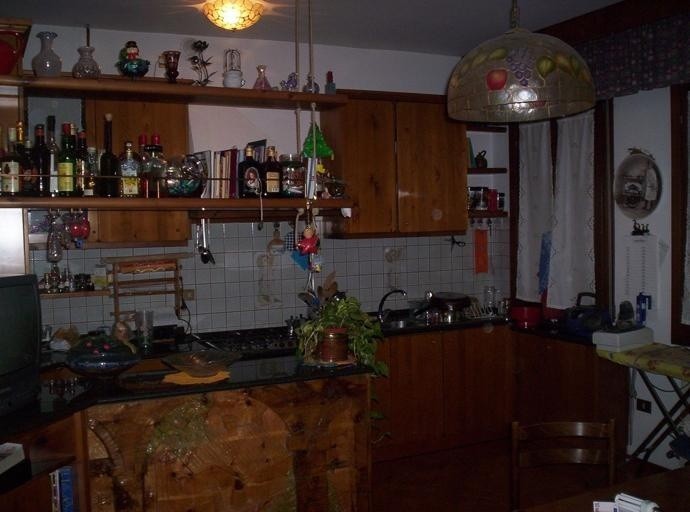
[371,318,416,329]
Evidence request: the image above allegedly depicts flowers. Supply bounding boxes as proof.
[191,40,216,86]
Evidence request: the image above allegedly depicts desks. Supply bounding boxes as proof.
[596,344,690,475]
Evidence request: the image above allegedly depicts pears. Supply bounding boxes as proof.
[536,56,557,80]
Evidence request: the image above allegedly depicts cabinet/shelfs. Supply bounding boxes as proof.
[322,89,468,236]
[512,332,627,454]
[372,321,510,465]
[468,127,508,218]
[1,20,350,300]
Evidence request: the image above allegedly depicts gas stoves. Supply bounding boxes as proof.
[209,334,299,358]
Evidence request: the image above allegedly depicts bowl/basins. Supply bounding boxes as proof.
[162,350,243,378]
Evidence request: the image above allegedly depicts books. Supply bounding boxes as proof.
[193,148,240,199]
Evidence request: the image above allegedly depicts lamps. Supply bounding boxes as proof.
[448,0,595,121]
[202,0,265,31]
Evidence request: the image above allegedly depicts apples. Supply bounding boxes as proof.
[487,68,507,90]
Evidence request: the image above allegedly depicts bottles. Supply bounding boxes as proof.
[424,302,464,324]
[42,263,92,294]
[635,291,647,327]
[466,184,506,212]
[1,113,169,199]
[483,285,506,317]
[236,144,305,199]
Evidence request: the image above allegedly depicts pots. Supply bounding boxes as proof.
[509,287,566,326]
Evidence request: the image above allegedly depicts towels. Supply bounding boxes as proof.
[475,230,488,272]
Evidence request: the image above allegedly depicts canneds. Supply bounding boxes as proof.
[321,328,348,362]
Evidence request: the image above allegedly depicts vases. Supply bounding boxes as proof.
[72,46,101,79]
[32,33,61,75]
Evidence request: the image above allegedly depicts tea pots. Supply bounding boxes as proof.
[475,150,488,168]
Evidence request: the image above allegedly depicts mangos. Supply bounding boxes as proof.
[552,53,577,80]
[470,50,489,69]
[457,59,473,78]
[487,45,507,62]
[570,53,583,78]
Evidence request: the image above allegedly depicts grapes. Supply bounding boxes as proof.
[503,44,536,87]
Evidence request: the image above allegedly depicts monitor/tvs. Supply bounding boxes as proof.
[0,272,41,414]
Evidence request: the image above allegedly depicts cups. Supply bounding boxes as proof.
[133,308,155,358]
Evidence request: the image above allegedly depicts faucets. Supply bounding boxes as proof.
[377,289,407,322]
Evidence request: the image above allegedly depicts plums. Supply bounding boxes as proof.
[84,340,92,348]
[103,343,111,351]
[92,348,100,355]
